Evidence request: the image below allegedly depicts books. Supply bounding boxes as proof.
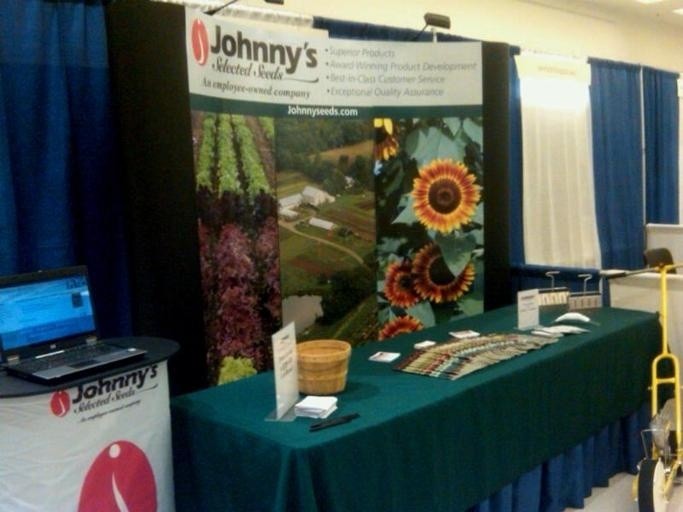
[391,333,560,382]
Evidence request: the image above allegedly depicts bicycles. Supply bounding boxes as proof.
[623,264,682,512]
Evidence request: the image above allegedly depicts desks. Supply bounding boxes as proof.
[167,300,672,512]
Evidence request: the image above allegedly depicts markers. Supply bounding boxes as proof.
[309,412,360,432]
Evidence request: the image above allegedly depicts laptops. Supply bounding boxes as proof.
[0,265,149,385]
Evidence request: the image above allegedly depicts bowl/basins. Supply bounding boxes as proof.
[296,339,352,396]
